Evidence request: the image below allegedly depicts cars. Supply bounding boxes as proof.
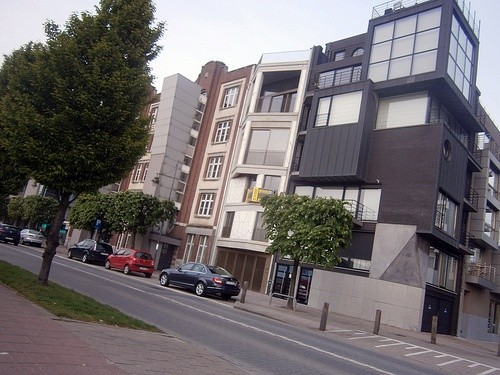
[159,262,241,300]
[66,238,113,264]
[0,223,20,246]
[19,228,44,246]
[105,248,154,278]
